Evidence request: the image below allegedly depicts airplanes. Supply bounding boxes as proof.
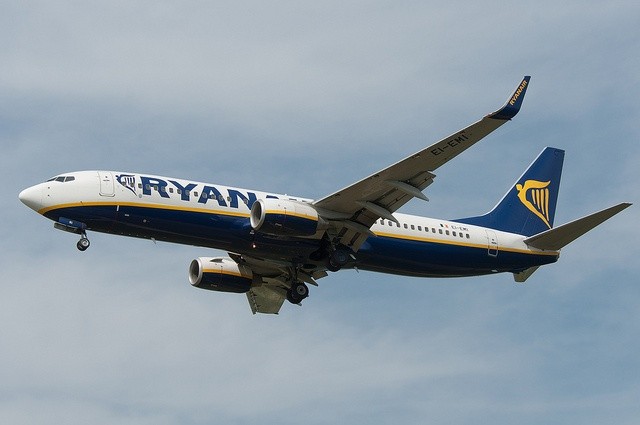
[16,74,634,316]
